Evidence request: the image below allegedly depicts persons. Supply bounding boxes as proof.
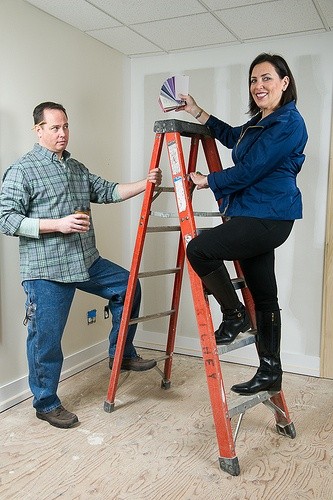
[175,53,308,395]
[0,101,163,429]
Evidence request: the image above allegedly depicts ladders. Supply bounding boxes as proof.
[103,119,296,477]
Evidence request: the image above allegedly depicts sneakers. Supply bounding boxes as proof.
[106,356,157,371]
[35,405,79,429]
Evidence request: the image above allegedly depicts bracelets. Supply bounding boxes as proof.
[195,109,204,119]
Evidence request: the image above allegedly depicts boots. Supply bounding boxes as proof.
[200,266,252,344]
[230,310,281,394]
